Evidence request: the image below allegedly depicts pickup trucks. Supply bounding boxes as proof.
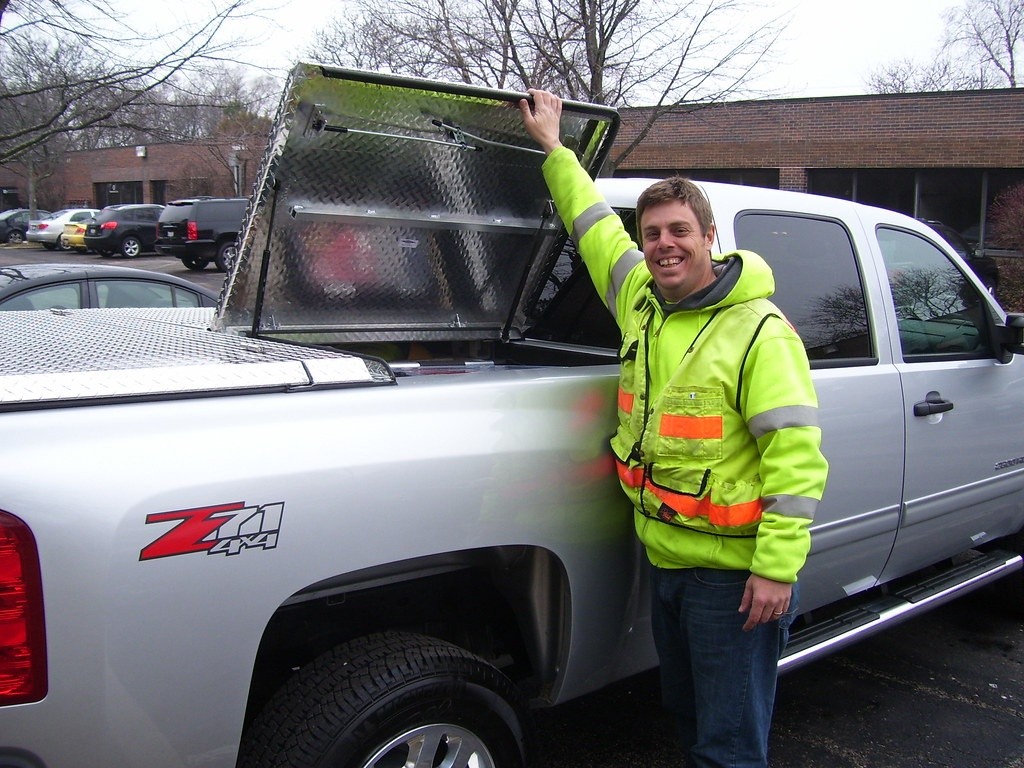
[0,60,1024,768]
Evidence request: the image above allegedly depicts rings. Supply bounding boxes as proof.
[774,611,783,615]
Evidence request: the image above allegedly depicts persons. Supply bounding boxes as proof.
[522,87,828,768]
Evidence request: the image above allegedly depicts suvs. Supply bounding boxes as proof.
[892,219,999,302]
[153,196,252,273]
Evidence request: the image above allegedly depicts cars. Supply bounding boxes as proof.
[0,208,51,245]
[60,218,96,254]
[0,265,220,311]
[26,208,101,251]
[83,203,165,260]
